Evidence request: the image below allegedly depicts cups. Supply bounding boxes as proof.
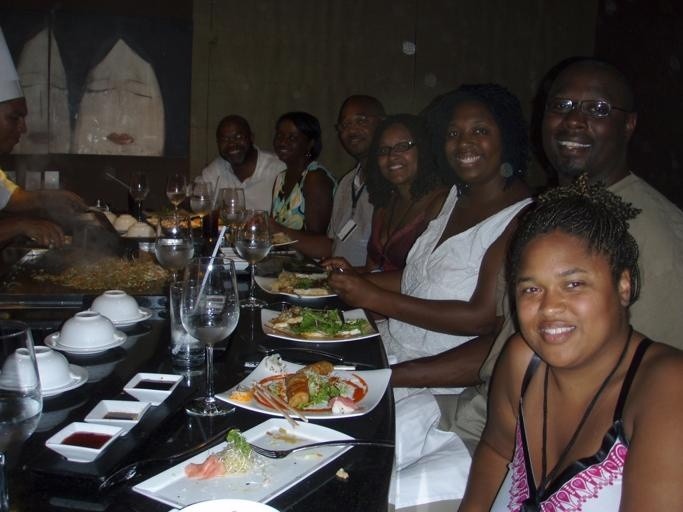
[166,173,186,211]
[180,256,240,417]
[156,214,195,282]
[188,178,212,245]
[169,280,207,371]
[218,187,246,246]
[129,171,149,219]
[230,209,268,308]
[1,318,44,512]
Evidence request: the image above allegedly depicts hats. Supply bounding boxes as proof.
[0,30,25,104]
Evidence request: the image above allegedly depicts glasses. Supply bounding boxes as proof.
[216,133,243,143]
[334,116,367,133]
[377,141,419,156]
[542,96,616,119]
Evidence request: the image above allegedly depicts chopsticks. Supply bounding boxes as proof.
[251,381,308,427]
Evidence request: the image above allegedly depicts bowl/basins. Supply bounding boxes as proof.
[115,214,136,231]
[127,223,155,236]
[122,371,183,407]
[58,310,118,348]
[83,366,117,384]
[101,209,113,223]
[120,336,139,350]
[224,262,250,271]
[84,399,151,438]
[120,338,137,351]
[2,346,72,392]
[91,289,141,321]
[45,422,126,463]
[34,413,69,434]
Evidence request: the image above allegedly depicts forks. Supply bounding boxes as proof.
[243,440,397,460]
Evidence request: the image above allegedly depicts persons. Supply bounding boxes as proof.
[320,84,534,364]
[387,58,683,512]
[193,114,287,219]
[0,28,87,250]
[352,113,452,274]
[457,173,683,511]
[269,111,337,234]
[251,95,386,267]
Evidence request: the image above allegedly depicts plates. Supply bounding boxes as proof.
[260,307,381,345]
[62,347,130,368]
[253,276,339,302]
[39,385,89,414]
[132,416,360,509]
[115,320,155,339]
[44,331,128,354]
[7,362,89,398]
[268,231,299,249]
[112,305,154,328]
[214,355,393,420]
